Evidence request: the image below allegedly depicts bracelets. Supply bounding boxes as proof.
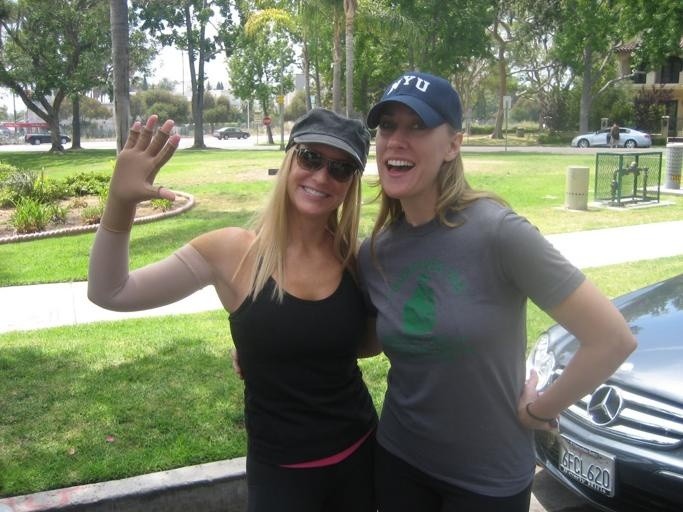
[526,403,553,421]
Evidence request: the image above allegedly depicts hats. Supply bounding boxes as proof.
[367,71,463,128]
[286,107,368,172]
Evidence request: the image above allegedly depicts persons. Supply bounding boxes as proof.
[88,108,378,511]
[232,73,635,510]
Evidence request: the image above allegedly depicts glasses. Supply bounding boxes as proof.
[297,147,358,182]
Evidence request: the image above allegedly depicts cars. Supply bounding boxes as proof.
[0,125,9,139]
[24,131,70,145]
[523,273,681,512]
[569,125,651,147]
[213,125,251,139]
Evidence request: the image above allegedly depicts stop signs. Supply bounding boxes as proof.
[262,116,272,125]
